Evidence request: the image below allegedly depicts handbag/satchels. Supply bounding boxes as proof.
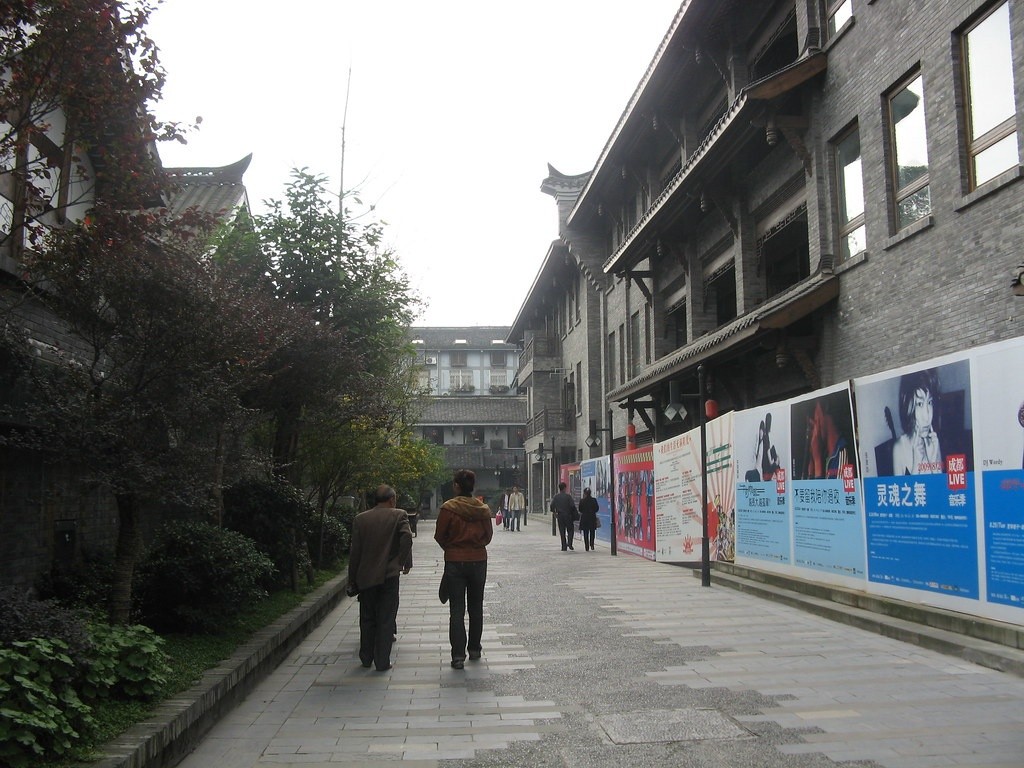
[595,518,601,528]
[496,510,502,525]
[439,571,448,604]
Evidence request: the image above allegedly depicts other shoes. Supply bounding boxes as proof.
[590,544,594,550]
[451,660,464,669]
[376,662,392,671]
[362,659,372,666]
[568,544,574,550]
[506,528,509,531]
[469,648,481,659]
[586,546,589,551]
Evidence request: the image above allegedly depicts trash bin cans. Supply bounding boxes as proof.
[407,513,418,537]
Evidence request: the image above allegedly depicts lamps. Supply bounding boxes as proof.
[585,428,610,447]
[534,449,554,461]
[662,394,699,427]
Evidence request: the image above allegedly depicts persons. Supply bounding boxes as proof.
[434,469,493,670]
[346,485,413,671]
[550,482,580,551]
[509,485,525,532]
[893,370,942,477]
[745,413,781,484]
[808,394,850,480]
[579,488,599,551]
[499,488,510,531]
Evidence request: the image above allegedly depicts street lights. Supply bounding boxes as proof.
[584,409,617,557]
[495,460,506,494]
[662,363,712,586]
[534,435,557,536]
[511,451,528,525]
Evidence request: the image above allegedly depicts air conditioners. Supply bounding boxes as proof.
[425,356,437,365]
